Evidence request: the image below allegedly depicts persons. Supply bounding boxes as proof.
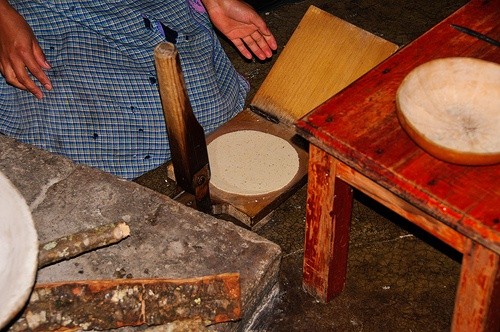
[0,0,278,182]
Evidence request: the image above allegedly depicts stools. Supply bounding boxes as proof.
[294,1,500,332]
